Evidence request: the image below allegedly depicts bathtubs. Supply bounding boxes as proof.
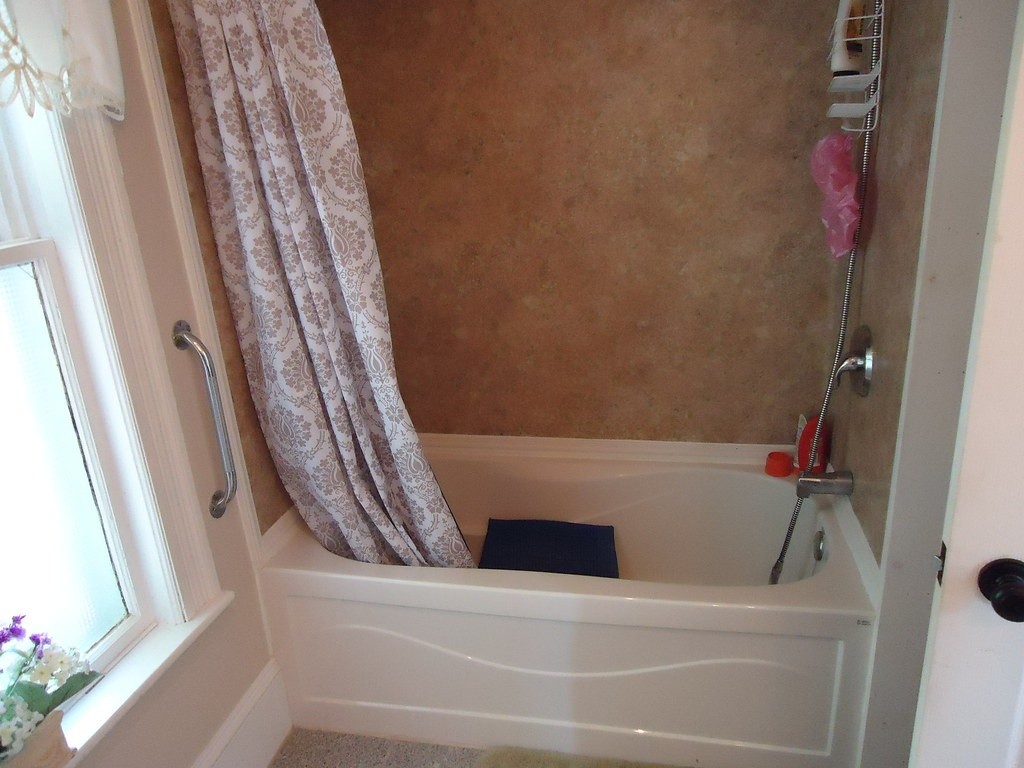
[266,431,878,768]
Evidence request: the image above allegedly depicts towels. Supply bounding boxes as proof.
[478,517,620,579]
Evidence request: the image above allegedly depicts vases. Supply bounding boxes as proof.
[0,708,79,767]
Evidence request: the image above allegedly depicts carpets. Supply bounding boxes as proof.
[473,746,699,768]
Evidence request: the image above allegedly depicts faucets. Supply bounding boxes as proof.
[796,470,853,498]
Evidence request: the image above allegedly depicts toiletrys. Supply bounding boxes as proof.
[763,413,828,477]
[830,0,867,77]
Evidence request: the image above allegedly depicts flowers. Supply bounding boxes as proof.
[0,614,102,763]
